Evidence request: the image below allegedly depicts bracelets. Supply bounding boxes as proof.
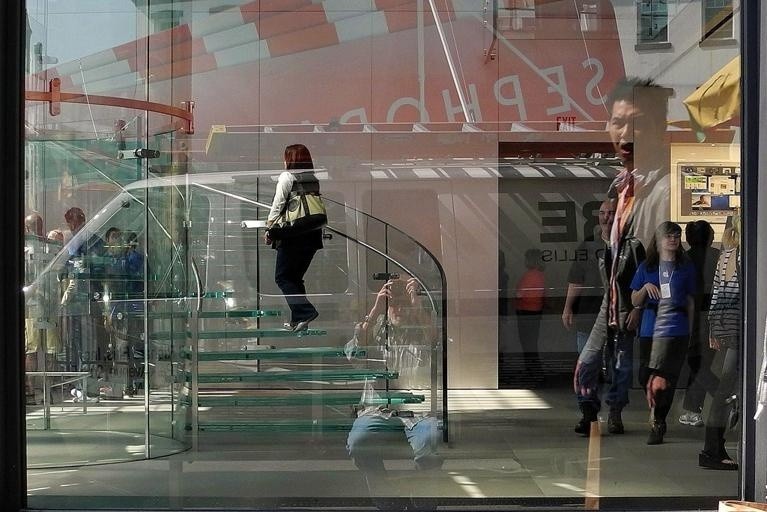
[265,228,270,232]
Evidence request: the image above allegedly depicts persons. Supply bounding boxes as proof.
[573,75,688,408]
[693,195,710,205]
[699,210,742,470]
[345,269,446,476]
[677,220,740,430]
[156,135,172,175]
[264,143,324,335]
[631,221,695,445]
[561,197,647,435]
[516,249,547,384]
[113,119,128,151]
[499,249,514,384]
[24,208,152,401]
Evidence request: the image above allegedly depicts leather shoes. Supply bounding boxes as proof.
[283,313,318,332]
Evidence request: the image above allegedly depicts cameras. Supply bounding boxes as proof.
[385,280,411,308]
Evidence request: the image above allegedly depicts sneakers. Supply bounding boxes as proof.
[575,400,738,471]
[71,387,122,404]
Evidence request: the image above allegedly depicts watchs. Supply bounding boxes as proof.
[363,315,378,325]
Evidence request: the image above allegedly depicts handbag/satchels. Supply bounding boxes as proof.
[284,192,327,231]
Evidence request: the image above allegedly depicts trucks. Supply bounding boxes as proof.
[23,164,627,391]
